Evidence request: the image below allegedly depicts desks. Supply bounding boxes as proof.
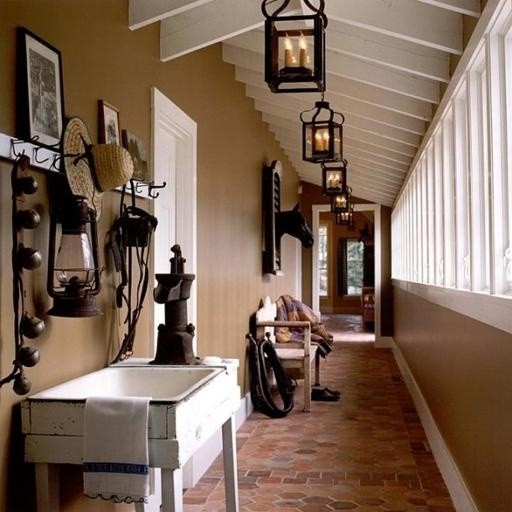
[21,357,241,512]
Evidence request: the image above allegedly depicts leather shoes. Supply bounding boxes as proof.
[312,387,340,395]
[311,388,340,401]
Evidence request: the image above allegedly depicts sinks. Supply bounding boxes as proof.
[21,364,241,470]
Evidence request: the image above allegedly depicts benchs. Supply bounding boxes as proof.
[255,296,321,413]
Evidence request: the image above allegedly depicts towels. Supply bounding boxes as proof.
[83,394,153,504]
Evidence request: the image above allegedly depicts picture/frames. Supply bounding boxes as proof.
[16,26,65,151]
[98,99,122,146]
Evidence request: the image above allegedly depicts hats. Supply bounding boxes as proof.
[62,117,135,223]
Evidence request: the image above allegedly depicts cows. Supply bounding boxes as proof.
[275,202,314,249]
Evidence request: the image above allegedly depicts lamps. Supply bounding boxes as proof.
[261,0,328,93]
[46,154,104,318]
[300,93,353,225]
